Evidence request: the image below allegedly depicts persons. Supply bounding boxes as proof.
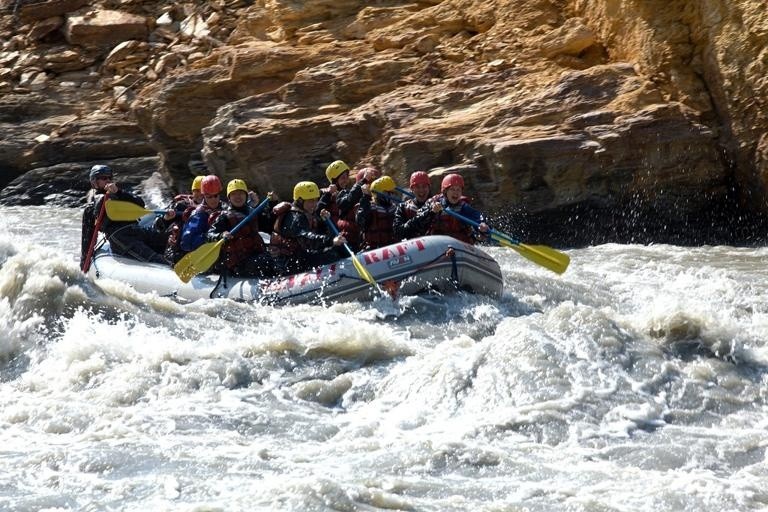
[139,158,490,281]
[81,165,170,273]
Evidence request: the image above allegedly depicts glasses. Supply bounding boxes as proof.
[98,176,112,181]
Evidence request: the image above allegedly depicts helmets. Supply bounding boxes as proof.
[89,165,113,177]
[293,161,464,201]
[227,179,248,197]
[192,176,205,190]
[201,175,222,195]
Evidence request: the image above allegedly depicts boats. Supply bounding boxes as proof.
[78,234,503,310]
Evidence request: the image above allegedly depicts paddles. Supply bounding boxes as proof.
[324,217,377,287]
[174,196,273,282]
[106,200,168,222]
[445,209,569,275]
